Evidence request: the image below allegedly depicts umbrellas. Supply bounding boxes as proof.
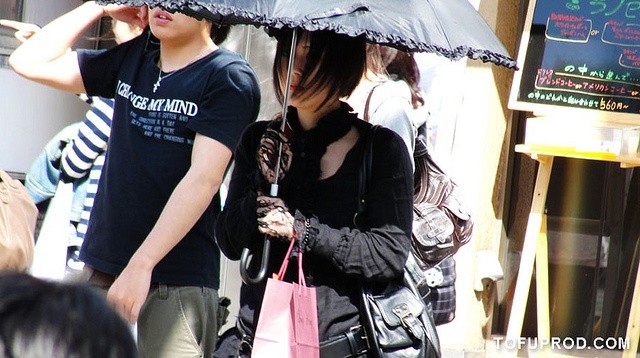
[99,0,522,289]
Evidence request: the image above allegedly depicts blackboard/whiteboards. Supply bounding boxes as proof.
[507,0,640,125]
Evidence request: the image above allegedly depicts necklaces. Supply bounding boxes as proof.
[153,43,214,91]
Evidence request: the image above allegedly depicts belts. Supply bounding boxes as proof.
[319,326,371,358]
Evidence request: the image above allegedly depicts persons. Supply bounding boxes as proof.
[0,2,149,286]
[338,40,426,180]
[215,25,416,358]
[0,271,140,357]
[7,1,262,357]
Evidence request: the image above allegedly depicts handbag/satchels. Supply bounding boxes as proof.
[0,167,37,273]
[250,232,319,358]
[364,79,474,270]
[356,123,441,357]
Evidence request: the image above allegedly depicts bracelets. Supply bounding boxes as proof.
[301,216,311,247]
